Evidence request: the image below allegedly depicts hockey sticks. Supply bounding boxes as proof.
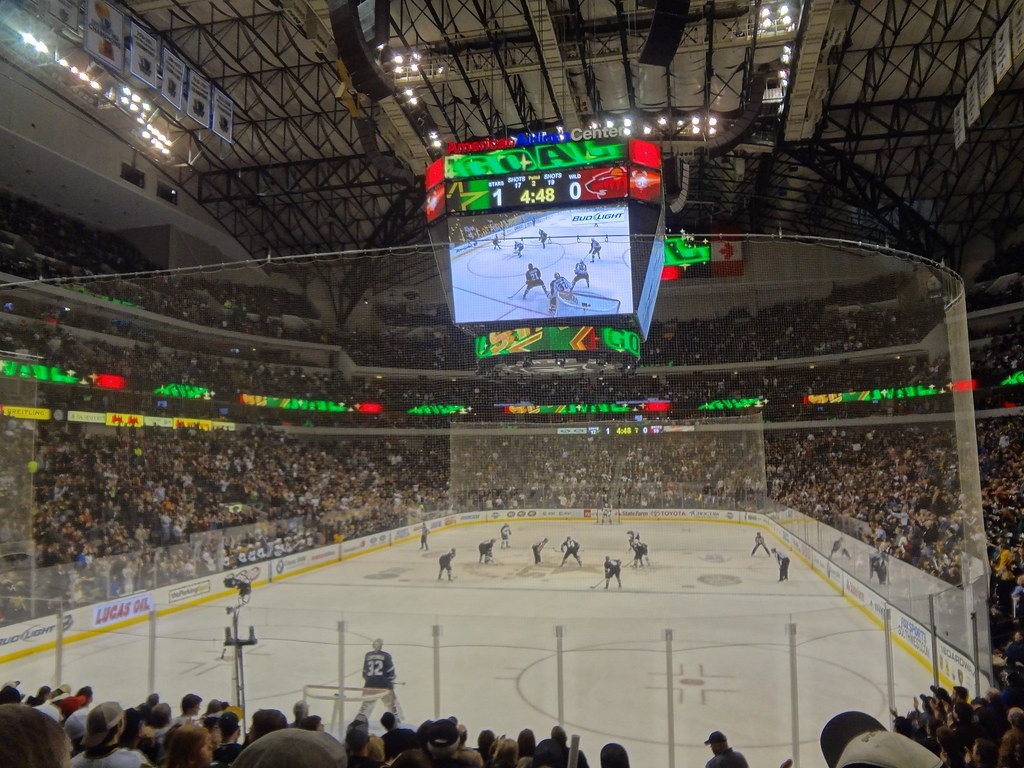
[622,560,634,567]
[392,681,406,685]
[553,546,585,553]
[589,578,606,589]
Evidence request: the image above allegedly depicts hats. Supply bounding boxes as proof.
[703,731,727,744]
[427,719,459,749]
[0,703,71,768]
[347,713,369,735]
[0,681,17,693]
[232,727,347,768]
[920,693,935,708]
[86,701,124,734]
[293,701,309,717]
[152,703,171,717]
[219,712,239,734]
[65,707,91,739]
[59,684,71,694]
[931,686,949,702]
[835,732,943,768]
[820,710,891,768]
[182,693,203,711]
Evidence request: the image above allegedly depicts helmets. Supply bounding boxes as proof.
[372,639,383,650]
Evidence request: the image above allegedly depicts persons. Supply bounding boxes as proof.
[360,639,405,724]
[479,539,496,564]
[501,523,511,549]
[449,203,628,314]
[0,191,588,768]
[601,503,612,523]
[532,538,549,565]
[604,556,622,588]
[420,523,431,550]
[560,536,583,567]
[627,531,644,566]
[632,539,650,567]
[831,538,851,560]
[568,272,1024,768]
[751,532,770,557]
[438,548,456,581]
[869,553,888,585]
[771,548,790,581]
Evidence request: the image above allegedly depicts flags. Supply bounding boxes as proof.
[711,225,744,277]
